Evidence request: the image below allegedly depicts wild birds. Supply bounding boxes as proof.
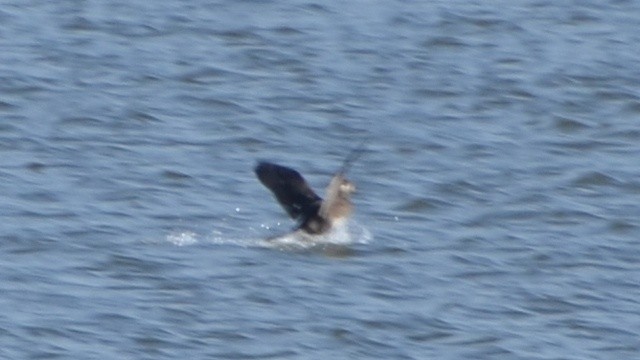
[254,134,369,243]
